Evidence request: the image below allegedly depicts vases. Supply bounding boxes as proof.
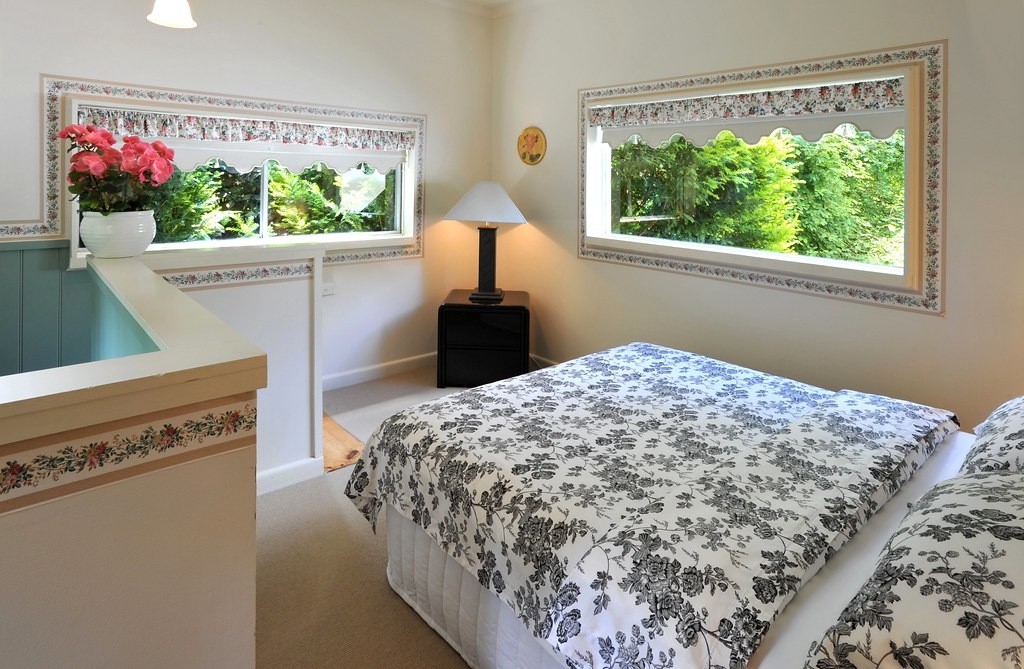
[80,210,158,259]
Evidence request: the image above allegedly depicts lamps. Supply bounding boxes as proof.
[444,180,527,300]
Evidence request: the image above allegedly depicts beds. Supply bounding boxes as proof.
[342,341,1024,669]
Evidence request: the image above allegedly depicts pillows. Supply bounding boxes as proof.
[804,471,1024,669]
[958,395,1024,471]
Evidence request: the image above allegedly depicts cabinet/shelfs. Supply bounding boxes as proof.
[437,287,531,388]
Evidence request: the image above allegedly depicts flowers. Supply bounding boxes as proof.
[57,124,175,216]
